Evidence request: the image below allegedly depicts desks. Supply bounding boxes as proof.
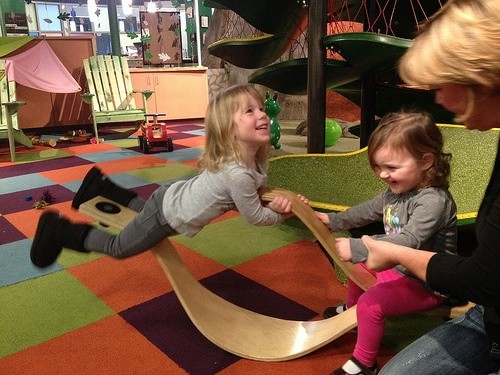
[129,66,208,122]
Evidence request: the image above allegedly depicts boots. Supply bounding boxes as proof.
[30,210,96,267]
[71,166,138,210]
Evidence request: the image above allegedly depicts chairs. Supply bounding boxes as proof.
[0,59,33,163]
[80,55,155,145]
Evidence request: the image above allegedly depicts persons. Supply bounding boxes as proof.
[361,0,500,375]
[30,85,308,267]
[315,113,458,375]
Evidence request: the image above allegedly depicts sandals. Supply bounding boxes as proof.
[332,356,377,375]
[323,304,347,318]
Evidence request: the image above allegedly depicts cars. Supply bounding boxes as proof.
[138,111,174,153]
